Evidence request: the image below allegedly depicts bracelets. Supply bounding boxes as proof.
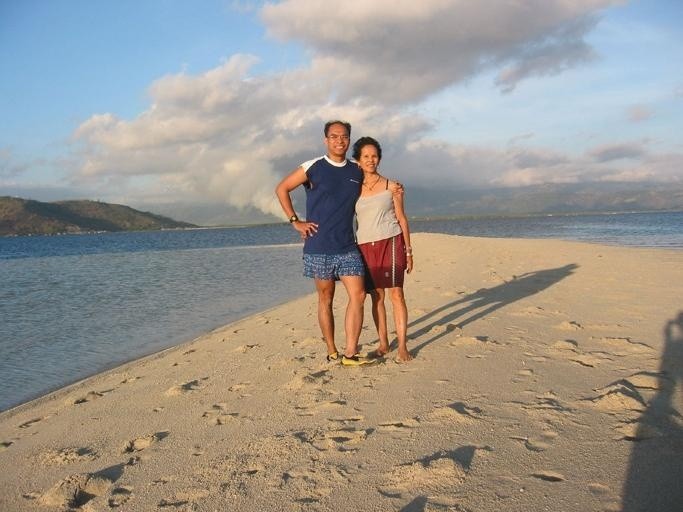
[406,252,413,256]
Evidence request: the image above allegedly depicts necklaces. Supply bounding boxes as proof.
[363,175,380,192]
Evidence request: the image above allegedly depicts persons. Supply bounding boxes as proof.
[275,119,405,366]
[352,136,415,362]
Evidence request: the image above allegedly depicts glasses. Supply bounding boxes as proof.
[328,135,349,141]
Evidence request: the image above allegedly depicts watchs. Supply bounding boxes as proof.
[289,214,298,223]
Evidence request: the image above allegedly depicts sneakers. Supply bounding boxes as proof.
[341,354,377,365]
[327,351,340,362]
